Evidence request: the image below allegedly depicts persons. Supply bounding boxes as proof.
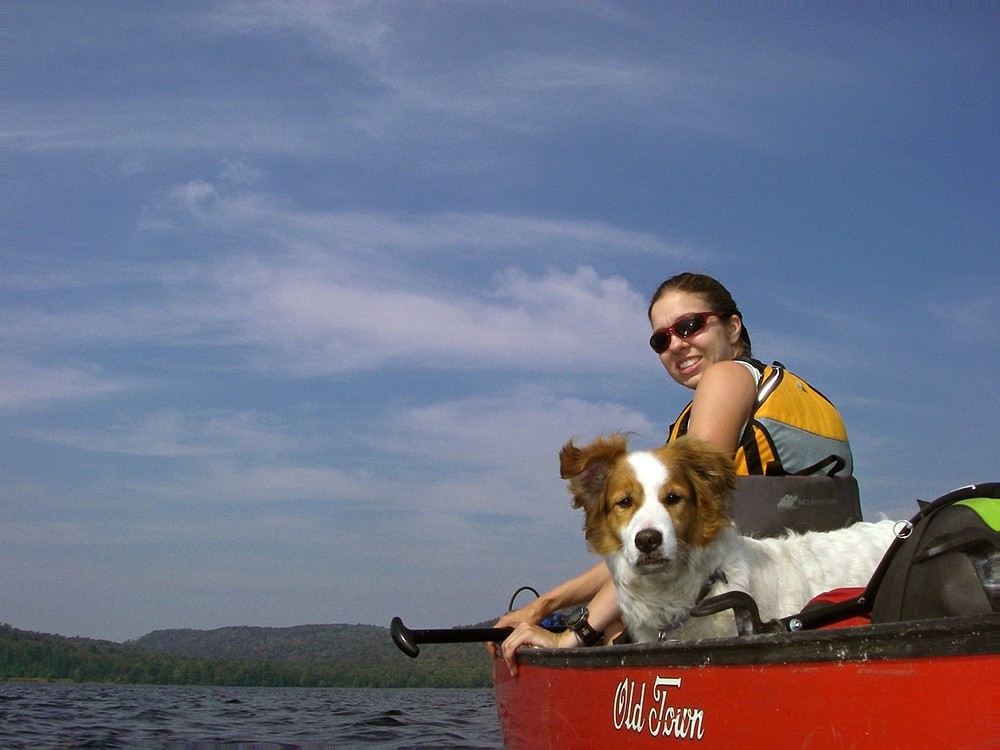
[487,271,864,680]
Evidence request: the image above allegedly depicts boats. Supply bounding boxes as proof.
[493,618,1000,748]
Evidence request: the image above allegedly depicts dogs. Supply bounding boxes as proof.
[558,427,911,646]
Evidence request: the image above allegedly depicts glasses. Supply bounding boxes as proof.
[650,311,727,355]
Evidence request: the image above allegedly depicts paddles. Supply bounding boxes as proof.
[390,616,571,659]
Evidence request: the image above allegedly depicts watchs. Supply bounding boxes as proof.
[566,607,599,646]
